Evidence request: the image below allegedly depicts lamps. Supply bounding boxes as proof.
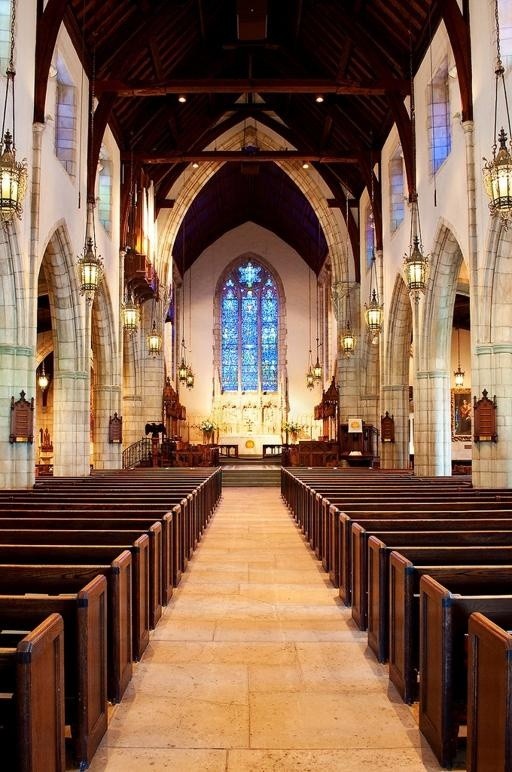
[75,48,105,303]
[400,32,430,303]
[147,298,163,358]
[454,327,465,389]
[480,0,512,231]
[37,331,49,391]
[306,338,323,390]
[178,228,195,389]
[0,1,29,236]
[336,192,357,359]
[363,165,385,336]
[119,299,142,338]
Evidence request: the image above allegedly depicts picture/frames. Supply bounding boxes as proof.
[450,388,472,441]
